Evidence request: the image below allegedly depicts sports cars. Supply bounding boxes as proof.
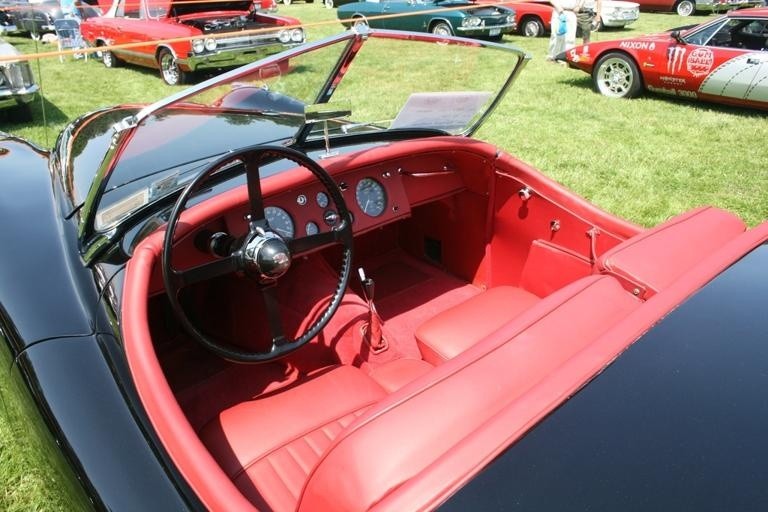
[0,26,768,512]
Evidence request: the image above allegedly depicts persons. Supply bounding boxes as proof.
[59,0,85,59]
[544,0,585,65]
[576,0,601,44]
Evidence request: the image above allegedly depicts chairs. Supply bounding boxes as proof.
[409,203,751,367]
[198,269,645,509]
[54,19,88,62]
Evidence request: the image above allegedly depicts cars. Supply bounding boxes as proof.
[554,8,768,112]
